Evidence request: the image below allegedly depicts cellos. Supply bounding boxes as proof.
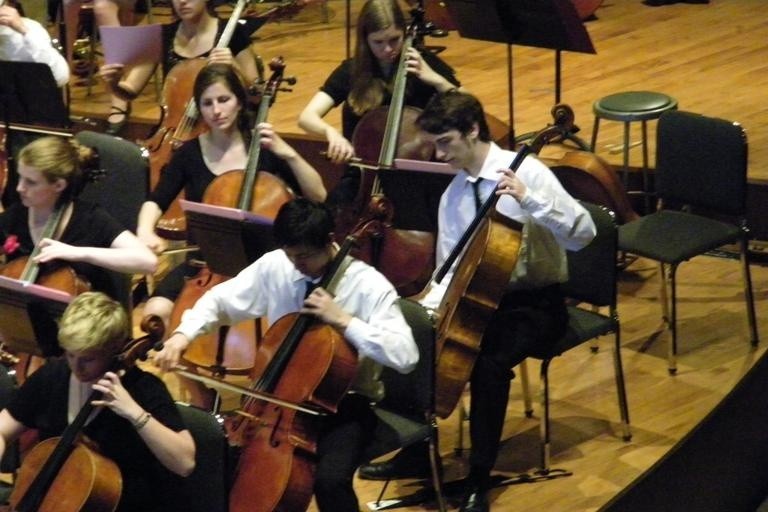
[223,194,393,510]
[334,1,448,297]
[9,315,164,512]
[548,150,642,226]
[436,104,579,419]
[2,170,109,390]
[163,55,296,371]
[143,1,250,231]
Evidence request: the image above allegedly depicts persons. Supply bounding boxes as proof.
[358,88,598,512]
[100,1,261,101]
[92,1,134,133]
[298,0,461,167]
[0,136,159,279]
[137,63,329,255]
[153,199,420,512]
[0,291,196,512]
[0,0,70,89]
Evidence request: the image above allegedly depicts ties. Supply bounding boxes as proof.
[305,280,319,299]
[471,177,483,211]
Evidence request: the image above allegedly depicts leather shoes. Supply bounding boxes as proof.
[103,99,131,133]
[359,442,442,479]
[456,483,488,512]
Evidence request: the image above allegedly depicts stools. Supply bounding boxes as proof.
[590,91,681,215]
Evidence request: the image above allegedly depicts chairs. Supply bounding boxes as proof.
[71,131,153,232]
[174,400,229,511]
[456,199,634,475]
[615,110,757,377]
[353,294,449,512]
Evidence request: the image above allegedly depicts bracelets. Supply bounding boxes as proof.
[133,410,145,424]
[132,413,151,431]
